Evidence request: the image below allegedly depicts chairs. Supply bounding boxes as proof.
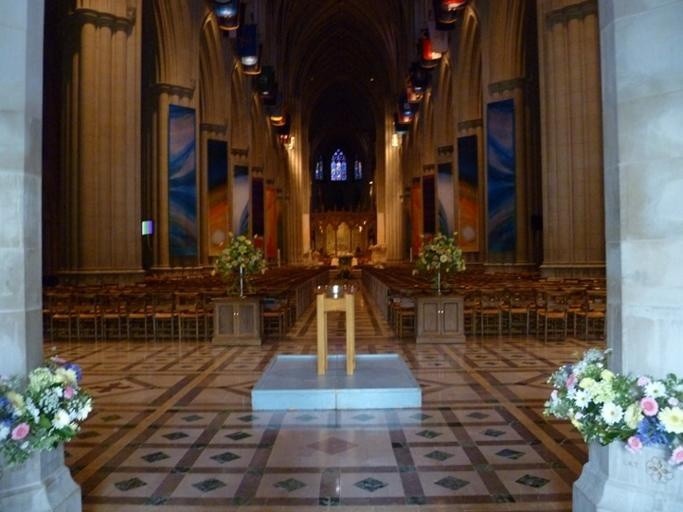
[41,264,328,346]
[361,266,607,344]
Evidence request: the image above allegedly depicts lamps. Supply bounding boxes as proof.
[390,0,468,147]
[214,0,296,151]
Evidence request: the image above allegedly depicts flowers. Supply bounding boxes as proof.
[213,232,267,276]
[542,347,683,472]
[0,356,93,465]
[416,231,466,273]
